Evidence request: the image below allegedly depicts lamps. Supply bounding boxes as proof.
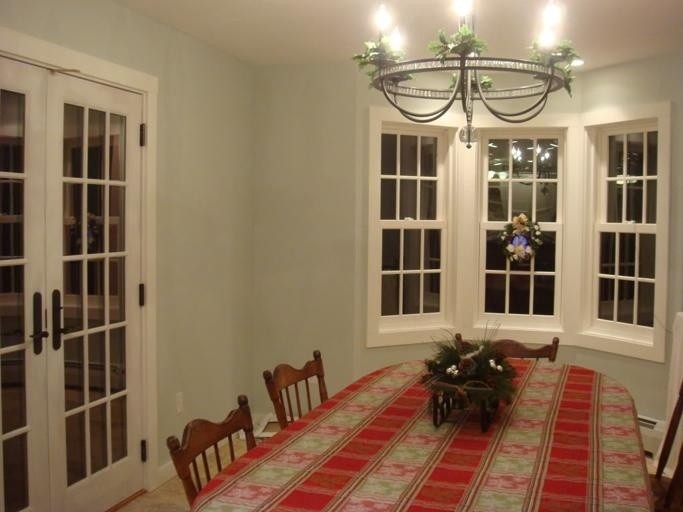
[350,0,589,149]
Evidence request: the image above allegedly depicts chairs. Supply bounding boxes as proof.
[452,334,561,363]
[265,350,329,429]
[167,394,258,506]
[646,383,683,512]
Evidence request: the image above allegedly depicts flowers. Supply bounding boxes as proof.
[502,212,542,265]
